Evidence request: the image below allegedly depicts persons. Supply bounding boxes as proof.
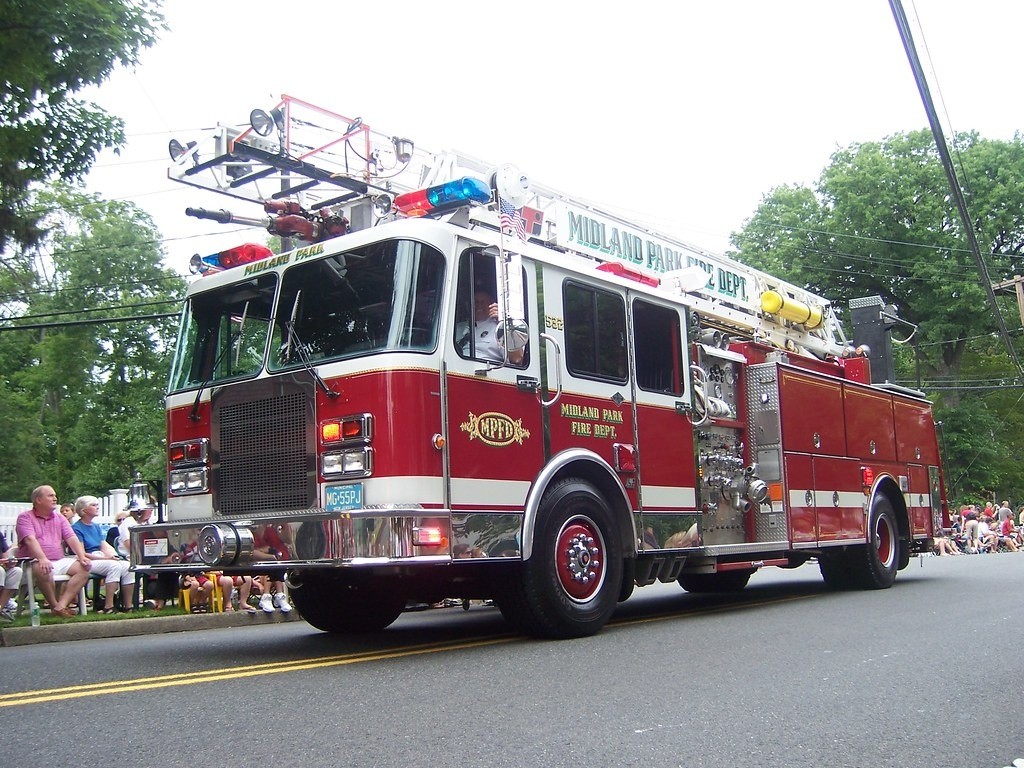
[184,573,292,613]
[108,498,179,613]
[143,523,294,563]
[664,523,698,547]
[0,532,23,615]
[921,501,1024,557]
[60,503,75,524]
[454,284,526,364]
[17,485,91,618]
[453,544,516,558]
[68,496,135,614]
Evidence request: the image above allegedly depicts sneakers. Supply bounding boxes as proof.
[274,592,293,612]
[259,594,276,612]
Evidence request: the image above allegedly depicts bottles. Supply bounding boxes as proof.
[32,602,40,627]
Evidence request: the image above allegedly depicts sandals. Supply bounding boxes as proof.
[198,601,207,613]
[191,601,200,613]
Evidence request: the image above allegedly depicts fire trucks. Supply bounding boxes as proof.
[128,93,957,639]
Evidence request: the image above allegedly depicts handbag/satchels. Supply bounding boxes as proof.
[966,512,976,520]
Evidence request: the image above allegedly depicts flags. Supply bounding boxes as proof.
[500,198,526,245]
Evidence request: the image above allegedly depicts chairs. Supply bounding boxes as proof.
[0,525,291,620]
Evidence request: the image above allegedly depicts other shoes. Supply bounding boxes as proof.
[1011,548,1019,552]
[3,597,138,618]
[143,599,157,608]
[1016,543,1022,547]
[957,552,965,555]
[950,551,957,555]
[940,551,951,557]
[955,546,959,549]
[959,549,962,552]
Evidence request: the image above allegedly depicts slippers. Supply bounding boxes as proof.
[154,601,163,612]
[224,606,235,613]
[238,605,257,613]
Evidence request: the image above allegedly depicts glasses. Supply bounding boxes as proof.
[462,551,473,554]
[117,518,125,522]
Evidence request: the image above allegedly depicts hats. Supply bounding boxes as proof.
[986,518,994,523]
